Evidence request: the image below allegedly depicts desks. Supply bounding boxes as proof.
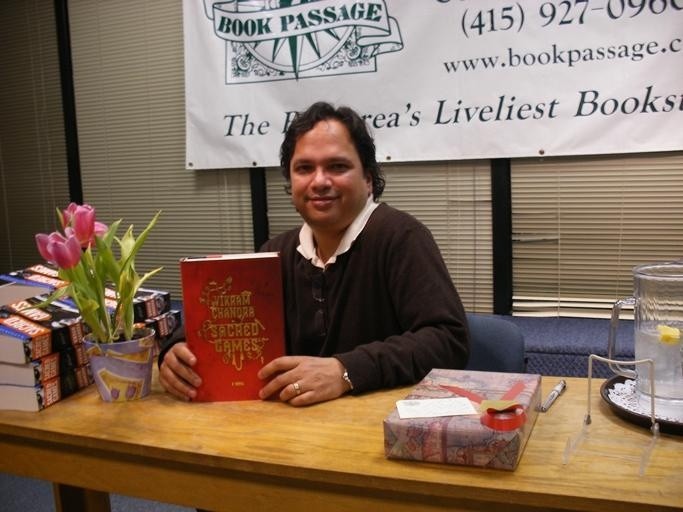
[0,357,683,512]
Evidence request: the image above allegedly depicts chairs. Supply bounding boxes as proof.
[462,313,527,375]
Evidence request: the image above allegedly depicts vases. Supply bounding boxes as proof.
[80,327,155,405]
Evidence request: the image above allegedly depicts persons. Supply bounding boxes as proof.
[155,104,470,407]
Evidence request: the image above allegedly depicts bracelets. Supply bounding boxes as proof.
[343,368,356,393]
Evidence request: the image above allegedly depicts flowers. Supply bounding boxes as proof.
[16,203,161,340]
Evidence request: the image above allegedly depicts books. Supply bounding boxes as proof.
[0,261,170,365]
[0,325,181,412]
[0,300,181,388]
[177,252,288,402]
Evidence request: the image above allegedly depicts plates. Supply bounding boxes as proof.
[598,370,683,436]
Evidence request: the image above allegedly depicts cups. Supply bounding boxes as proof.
[605,260,683,418]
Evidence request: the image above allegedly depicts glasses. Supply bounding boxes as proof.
[310,271,330,340]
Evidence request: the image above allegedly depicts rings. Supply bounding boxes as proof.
[292,382,300,394]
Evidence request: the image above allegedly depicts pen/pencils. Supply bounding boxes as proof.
[542,380,566,412]
